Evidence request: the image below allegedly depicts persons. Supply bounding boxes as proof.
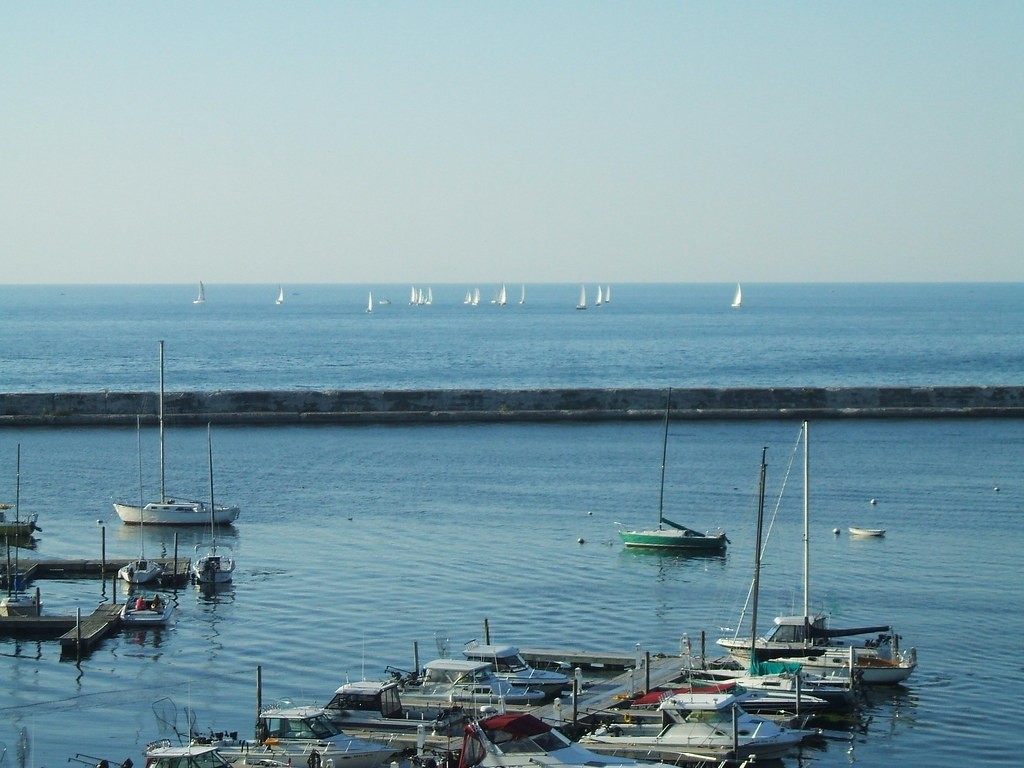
[816,635,833,647]
[394,672,423,686]
[214,562,219,569]
[136,593,162,611]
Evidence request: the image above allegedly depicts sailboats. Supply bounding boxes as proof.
[615,386,731,550]
[193,281,207,305]
[732,283,743,308]
[191,422,236,582]
[119,415,163,583]
[275,287,285,305]
[365,292,374,315]
[0,444,44,617]
[0,444,43,536]
[112,340,241,525]
[716,420,917,683]
[408,285,611,310]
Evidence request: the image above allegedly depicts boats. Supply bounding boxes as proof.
[383,658,545,705]
[848,527,885,536]
[630,669,830,720]
[120,597,174,627]
[459,713,682,768]
[191,705,402,768]
[679,660,854,699]
[67,740,234,768]
[293,681,464,737]
[463,638,570,698]
[579,694,802,758]
